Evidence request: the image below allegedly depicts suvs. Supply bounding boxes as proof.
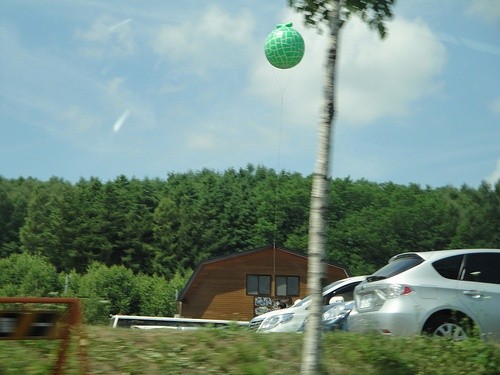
[351,248,499,344]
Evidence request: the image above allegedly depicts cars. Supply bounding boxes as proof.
[249,274,366,338]
